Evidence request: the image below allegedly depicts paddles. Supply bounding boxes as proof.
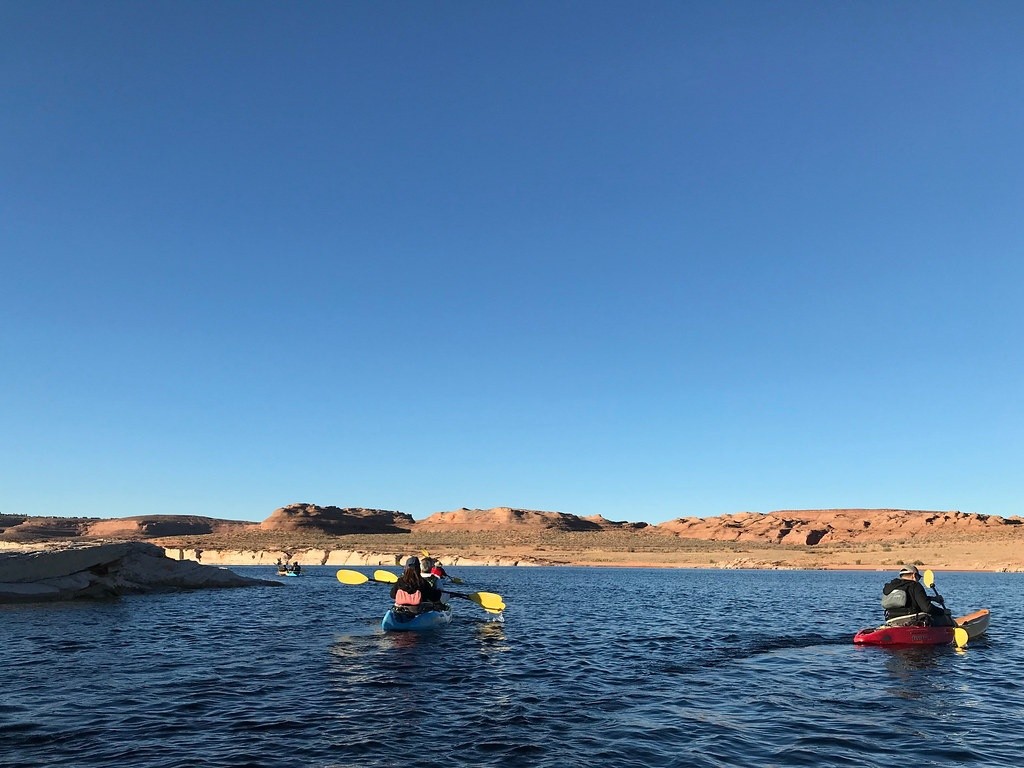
[923,569,970,648]
[336,569,507,614]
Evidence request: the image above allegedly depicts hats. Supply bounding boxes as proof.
[406,556,420,566]
[899,565,923,577]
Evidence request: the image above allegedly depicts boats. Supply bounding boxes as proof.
[380,602,453,631]
[853,608,991,647]
[277,570,298,577]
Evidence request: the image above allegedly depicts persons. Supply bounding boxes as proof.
[276,559,301,574]
[390,557,447,612]
[883,566,958,626]
